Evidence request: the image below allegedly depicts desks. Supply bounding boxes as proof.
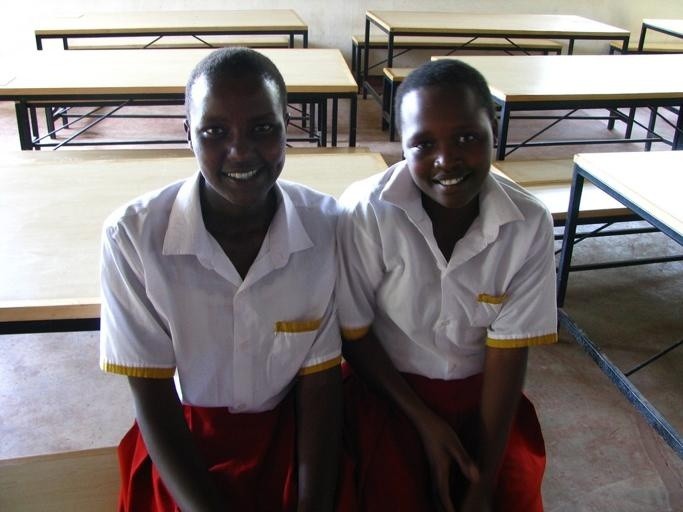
[556,150,682,457]
[0,151,390,336]
[361,10,681,149]
[1,10,358,150]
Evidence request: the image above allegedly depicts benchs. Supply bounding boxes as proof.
[496,157,645,255]
[351,34,561,80]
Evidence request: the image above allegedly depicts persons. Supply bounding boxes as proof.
[95,46,344,512]
[339,56,558,511]
